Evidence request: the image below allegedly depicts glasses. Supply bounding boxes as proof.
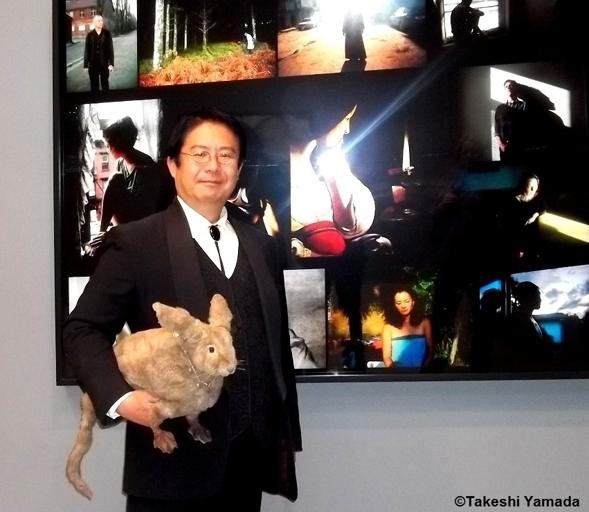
[177,151,242,165]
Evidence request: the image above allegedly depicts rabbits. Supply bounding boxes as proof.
[64,293,245,500]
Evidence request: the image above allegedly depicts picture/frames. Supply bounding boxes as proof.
[50,0,588,386]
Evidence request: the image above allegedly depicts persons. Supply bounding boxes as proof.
[506,280,589,370]
[244,32,256,53]
[446,0,492,41]
[80,11,115,98]
[495,79,535,165]
[339,6,367,62]
[289,98,375,259]
[379,285,436,371]
[60,107,306,512]
[93,120,162,237]
